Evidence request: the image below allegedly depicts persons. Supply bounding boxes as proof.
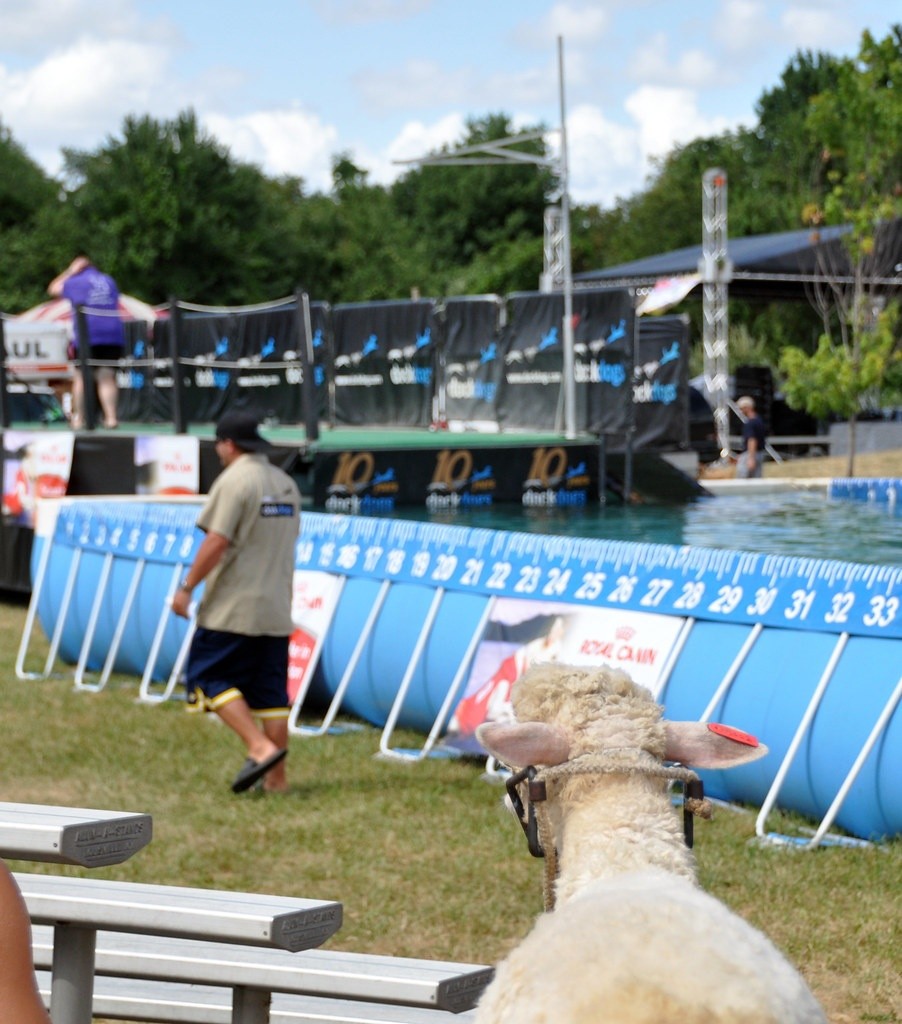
[47,253,126,429]
[172,409,303,802]
[0,859,53,1024]
[733,396,767,479]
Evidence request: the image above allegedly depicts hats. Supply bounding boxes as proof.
[217,410,270,449]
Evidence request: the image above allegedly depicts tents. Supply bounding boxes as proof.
[560,211,902,304]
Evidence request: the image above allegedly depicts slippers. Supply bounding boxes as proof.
[231,749,288,794]
[247,778,265,794]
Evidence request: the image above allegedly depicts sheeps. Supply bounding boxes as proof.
[473,661,834,1024]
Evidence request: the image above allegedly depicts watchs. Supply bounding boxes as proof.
[180,580,192,592]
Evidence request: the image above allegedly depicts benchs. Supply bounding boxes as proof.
[0,802,496,1024]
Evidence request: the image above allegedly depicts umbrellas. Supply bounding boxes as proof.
[15,290,170,321]
